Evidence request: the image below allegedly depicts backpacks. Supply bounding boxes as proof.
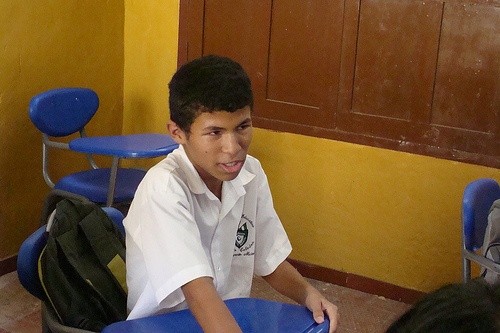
[35,188,131,332]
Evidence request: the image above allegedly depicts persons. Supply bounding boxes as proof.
[384,275,500,333]
[122,55,339,333]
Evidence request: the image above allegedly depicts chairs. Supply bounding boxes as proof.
[461,177,499,287]
[28,87,180,229]
[15,206,126,333]
